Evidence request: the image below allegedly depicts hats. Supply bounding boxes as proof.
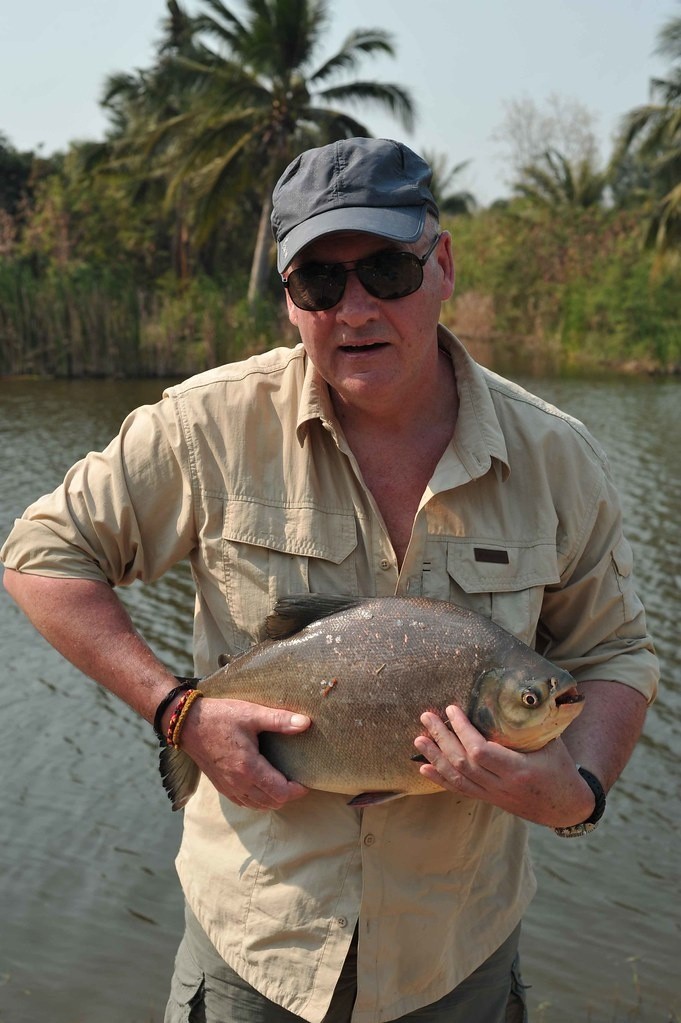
[270,137,439,274]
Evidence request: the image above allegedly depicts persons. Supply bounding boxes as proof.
[1,137,661,1023]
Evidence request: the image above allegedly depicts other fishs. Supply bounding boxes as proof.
[156,590,587,812]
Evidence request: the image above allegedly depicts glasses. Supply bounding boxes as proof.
[281,234,440,312]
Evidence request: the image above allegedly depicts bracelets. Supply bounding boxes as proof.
[167,690,203,751]
[555,766,606,838]
[153,682,194,740]
